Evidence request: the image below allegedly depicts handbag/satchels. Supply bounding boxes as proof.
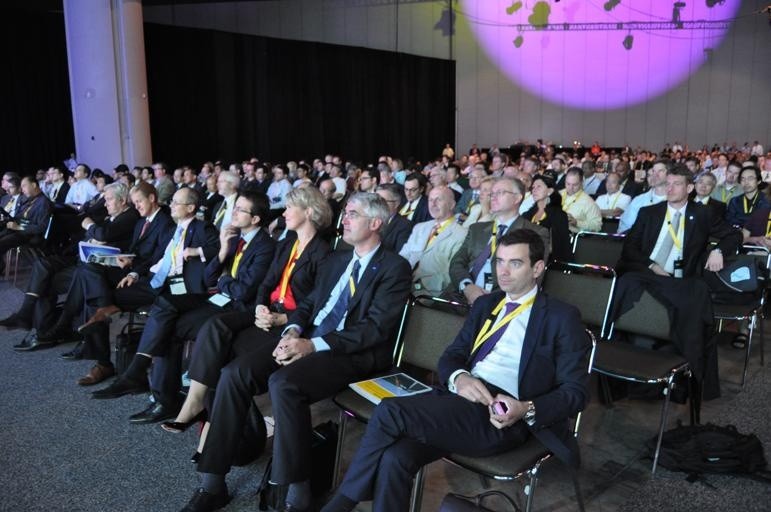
[258,421,341,511]
[707,252,759,296]
[437,491,520,512]
[115,321,154,377]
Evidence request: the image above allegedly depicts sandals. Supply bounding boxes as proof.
[732,328,751,350]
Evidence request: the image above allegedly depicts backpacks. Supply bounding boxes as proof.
[643,420,771,491]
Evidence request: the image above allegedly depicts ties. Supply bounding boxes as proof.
[654,210,683,270]
[214,201,228,230]
[309,259,363,341]
[428,222,443,242]
[470,225,508,281]
[403,199,414,219]
[138,220,150,241]
[149,226,184,292]
[237,240,246,254]
[468,302,521,371]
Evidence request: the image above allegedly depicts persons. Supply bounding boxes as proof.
[0,140,770,512]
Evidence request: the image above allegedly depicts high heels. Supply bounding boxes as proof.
[161,406,210,433]
[189,448,203,464]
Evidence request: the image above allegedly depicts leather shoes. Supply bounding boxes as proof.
[89,370,152,400]
[78,305,121,336]
[179,483,230,512]
[279,501,316,512]
[0,312,36,330]
[127,400,181,426]
[75,360,116,384]
[35,323,73,343]
[14,329,59,352]
[62,337,101,360]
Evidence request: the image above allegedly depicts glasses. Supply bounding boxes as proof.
[234,206,252,215]
[170,199,192,206]
[488,190,519,197]
[340,211,375,220]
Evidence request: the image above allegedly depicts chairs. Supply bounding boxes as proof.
[407,319,597,512]
[570,272,701,477]
[539,258,619,406]
[7,214,54,288]
[309,293,410,496]
[127,306,150,336]
[572,229,628,273]
[331,293,471,512]
[602,218,621,234]
[699,240,771,388]
